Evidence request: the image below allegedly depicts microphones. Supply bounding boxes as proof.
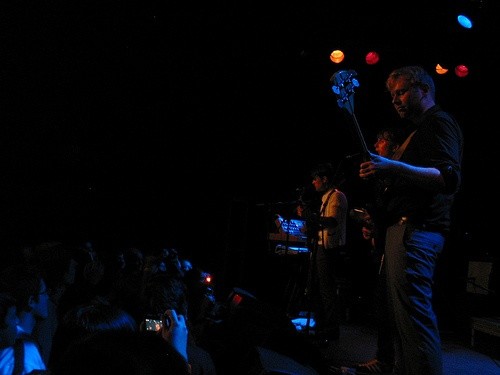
[296,184,314,191]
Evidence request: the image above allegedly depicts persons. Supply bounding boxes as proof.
[297,154,350,347]
[353,126,404,374]
[0,234,266,375]
[359,66,463,375]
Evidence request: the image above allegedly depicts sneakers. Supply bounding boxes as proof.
[340,357,388,375]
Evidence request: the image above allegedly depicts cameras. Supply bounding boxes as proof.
[143,314,171,335]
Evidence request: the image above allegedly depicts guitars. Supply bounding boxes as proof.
[328,69,397,204]
